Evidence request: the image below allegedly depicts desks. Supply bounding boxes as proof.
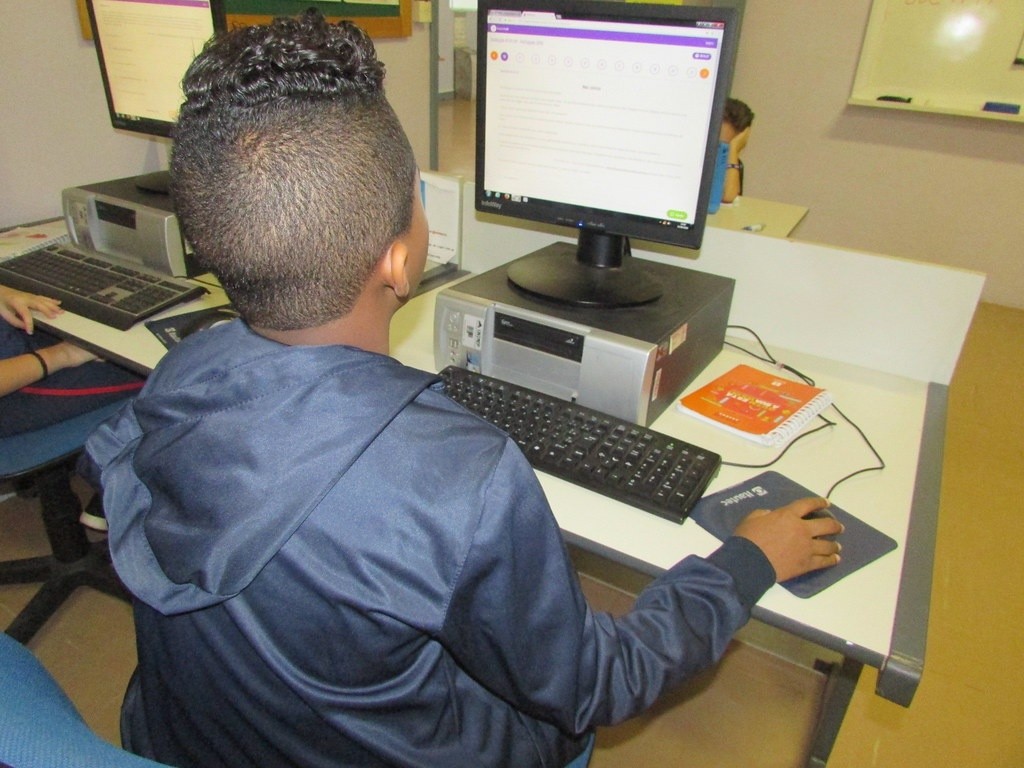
[0,184,986,768]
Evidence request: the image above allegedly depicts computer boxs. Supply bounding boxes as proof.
[432,241,736,429]
[63,172,212,280]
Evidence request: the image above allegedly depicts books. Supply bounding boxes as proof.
[677,364,832,450]
[0,227,70,263]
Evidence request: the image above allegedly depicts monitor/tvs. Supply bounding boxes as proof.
[85,0,228,193]
[473,0,747,310]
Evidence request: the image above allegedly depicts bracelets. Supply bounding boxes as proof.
[725,164,742,170]
[28,351,48,380]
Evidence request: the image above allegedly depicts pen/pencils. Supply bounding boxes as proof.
[742,224,766,232]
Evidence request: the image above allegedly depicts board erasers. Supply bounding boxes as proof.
[982,102,1021,115]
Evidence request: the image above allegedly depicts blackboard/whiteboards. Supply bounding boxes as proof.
[848,0,1024,124]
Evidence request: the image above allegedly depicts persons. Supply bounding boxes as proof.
[713,96,756,204]
[82,8,847,768]
[0,283,146,531]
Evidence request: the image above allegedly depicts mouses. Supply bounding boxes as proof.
[180,308,243,342]
[801,508,839,544]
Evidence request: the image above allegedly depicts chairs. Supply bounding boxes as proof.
[0,393,136,643]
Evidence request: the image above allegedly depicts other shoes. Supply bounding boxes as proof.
[79,493,110,530]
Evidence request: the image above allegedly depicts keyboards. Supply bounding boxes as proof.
[434,365,723,526]
[0,242,212,332]
